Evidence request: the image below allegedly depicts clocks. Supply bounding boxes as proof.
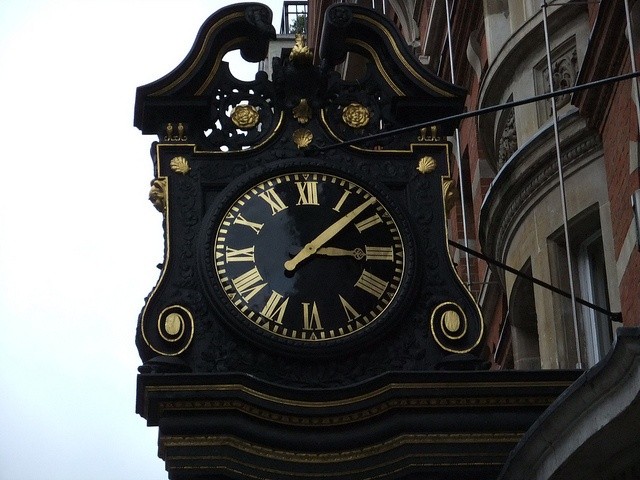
[134,1,492,373]
